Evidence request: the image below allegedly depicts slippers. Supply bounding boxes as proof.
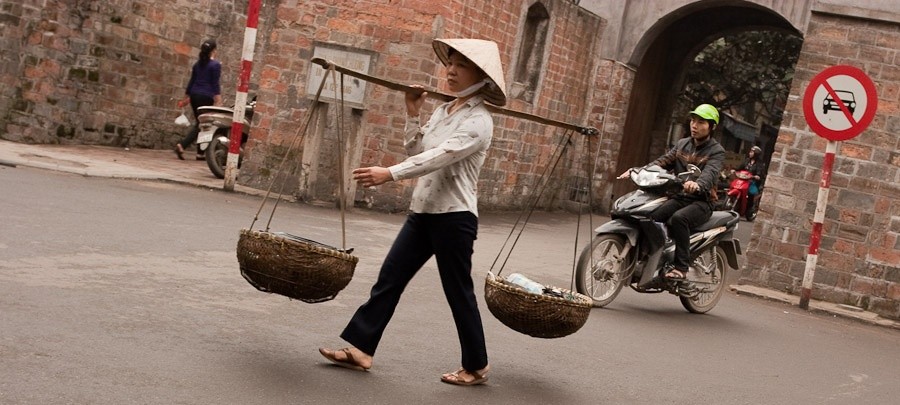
[196,154,207,160]
[441,367,490,386]
[319,344,366,372]
[174,146,185,160]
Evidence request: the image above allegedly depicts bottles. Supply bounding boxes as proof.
[507,273,545,294]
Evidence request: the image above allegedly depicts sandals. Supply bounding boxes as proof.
[667,269,689,282]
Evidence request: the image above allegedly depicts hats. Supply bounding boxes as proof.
[431,38,508,107]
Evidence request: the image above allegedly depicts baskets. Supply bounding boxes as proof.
[486,276,592,338]
[237,228,357,303]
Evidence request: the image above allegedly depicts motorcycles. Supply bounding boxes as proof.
[727,164,765,226]
[198,99,255,180]
[575,164,741,314]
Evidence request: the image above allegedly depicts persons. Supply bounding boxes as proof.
[730,146,763,222]
[175,39,223,161]
[621,103,726,281]
[319,38,506,385]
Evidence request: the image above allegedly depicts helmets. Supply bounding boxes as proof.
[751,145,762,155]
[688,104,720,125]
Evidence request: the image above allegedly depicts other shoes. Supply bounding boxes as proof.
[747,204,755,210]
